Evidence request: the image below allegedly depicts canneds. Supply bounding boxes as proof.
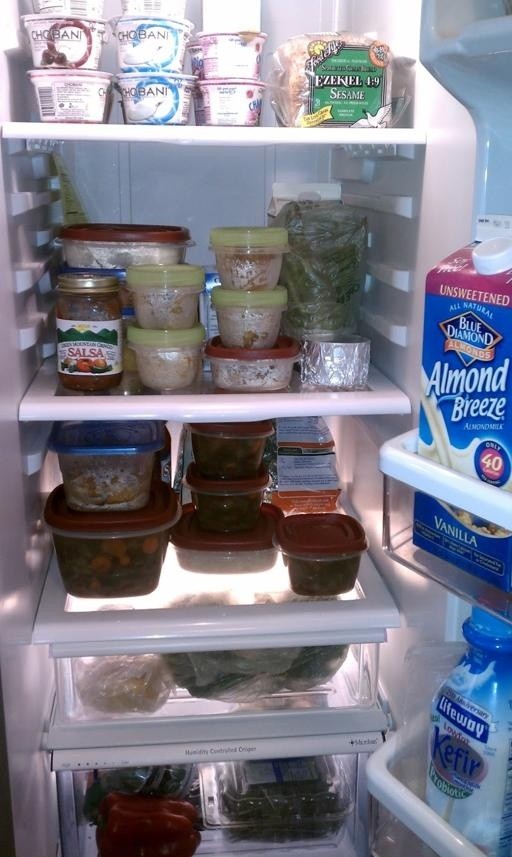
[54,274,122,390]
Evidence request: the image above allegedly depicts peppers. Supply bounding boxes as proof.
[90,792,202,857]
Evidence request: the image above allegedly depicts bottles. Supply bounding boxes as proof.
[431,603,511,851]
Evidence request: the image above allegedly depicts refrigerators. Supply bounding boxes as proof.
[1,0,511,849]
